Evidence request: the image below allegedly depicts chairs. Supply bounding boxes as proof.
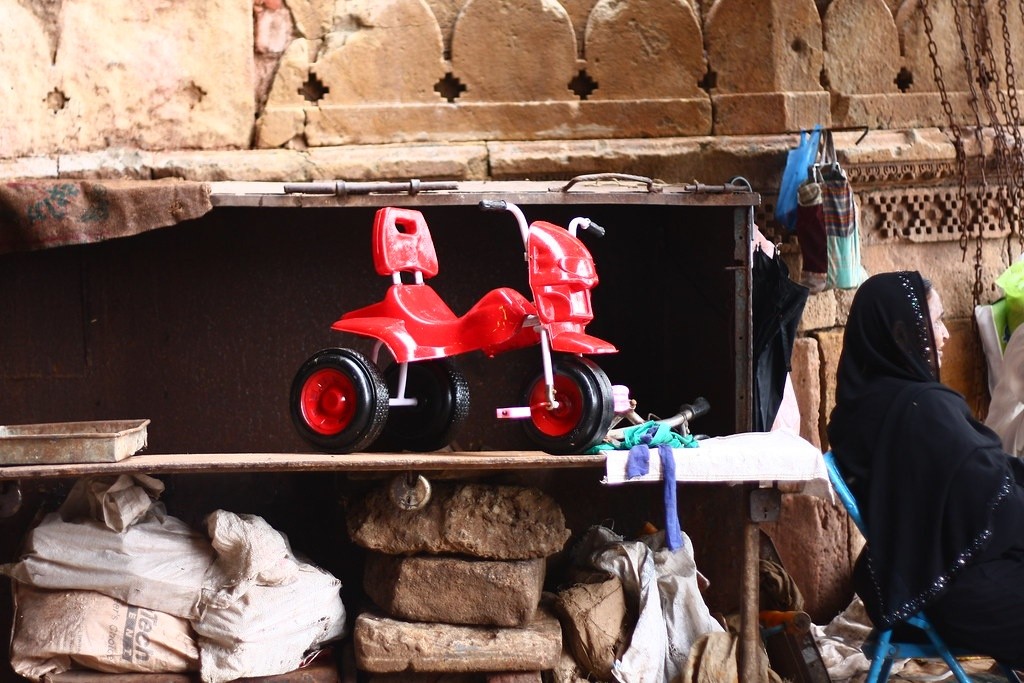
[825,450,974,682]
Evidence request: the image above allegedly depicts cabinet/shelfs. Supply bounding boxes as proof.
[0,180,762,683]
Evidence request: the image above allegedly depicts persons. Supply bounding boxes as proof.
[828,270,1024,683]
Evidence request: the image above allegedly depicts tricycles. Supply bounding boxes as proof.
[291,197,619,455]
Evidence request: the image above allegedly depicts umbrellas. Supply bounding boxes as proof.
[730,175,808,435]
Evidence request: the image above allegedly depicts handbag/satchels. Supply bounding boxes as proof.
[776,125,823,231]
[817,128,867,293]
[974,251,1024,459]
